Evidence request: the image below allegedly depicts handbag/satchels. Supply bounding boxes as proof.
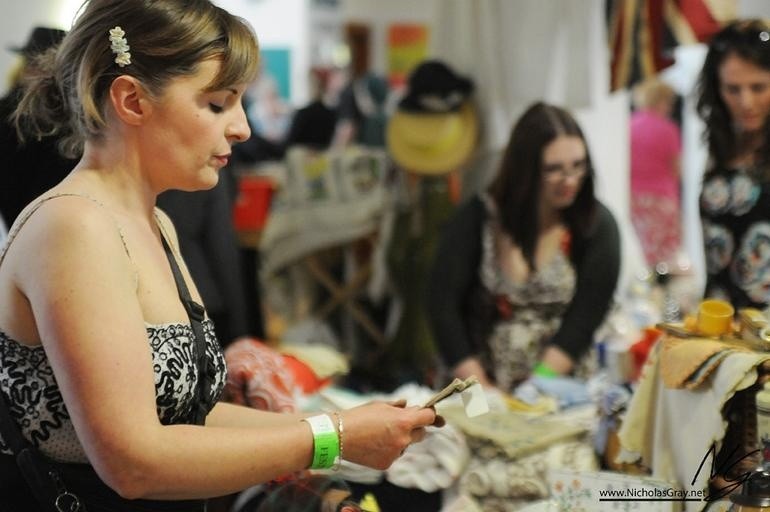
[16,447,207,511]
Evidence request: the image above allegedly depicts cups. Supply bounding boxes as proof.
[699,300,734,339]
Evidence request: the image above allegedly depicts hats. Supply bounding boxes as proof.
[396,60,477,113]
[9,26,66,58]
[388,105,478,174]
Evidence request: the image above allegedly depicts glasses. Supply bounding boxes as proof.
[713,25,770,47]
[543,161,593,184]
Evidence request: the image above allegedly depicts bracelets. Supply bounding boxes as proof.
[333,412,344,472]
[300,415,339,471]
[530,365,557,379]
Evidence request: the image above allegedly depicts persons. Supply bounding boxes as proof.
[328,50,390,150]
[0,28,81,231]
[290,67,336,152]
[427,102,621,391]
[696,19,770,317]
[0,0,447,511]
[156,175,248,344]
